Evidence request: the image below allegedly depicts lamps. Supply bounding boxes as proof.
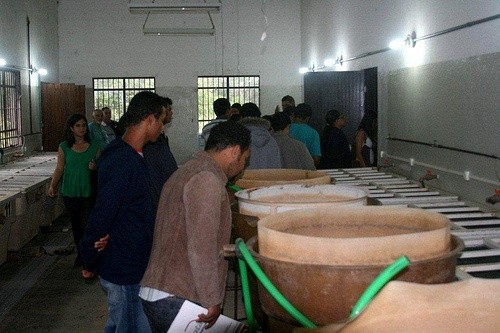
[127,0,222,35]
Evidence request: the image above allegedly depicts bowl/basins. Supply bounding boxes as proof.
[229,167,465,322]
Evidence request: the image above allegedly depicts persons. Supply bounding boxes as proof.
[229,103,241,117]
[281,95,296,109]
[50,113,104,280]
[288,104,322,168]
[145,96,179,214]
[261,113,273,134]
[201,97,231,145]
[78,91,166,333]
[355,111,378,168]
[283,106,296,122]
[102,107,119,131]
[270,110,316,171]
[236,102,283,168]
[86,109,108,147]
[319,110,354,169]
[138,120,252,333]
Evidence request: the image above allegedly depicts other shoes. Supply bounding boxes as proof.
[82,270,94,279]
